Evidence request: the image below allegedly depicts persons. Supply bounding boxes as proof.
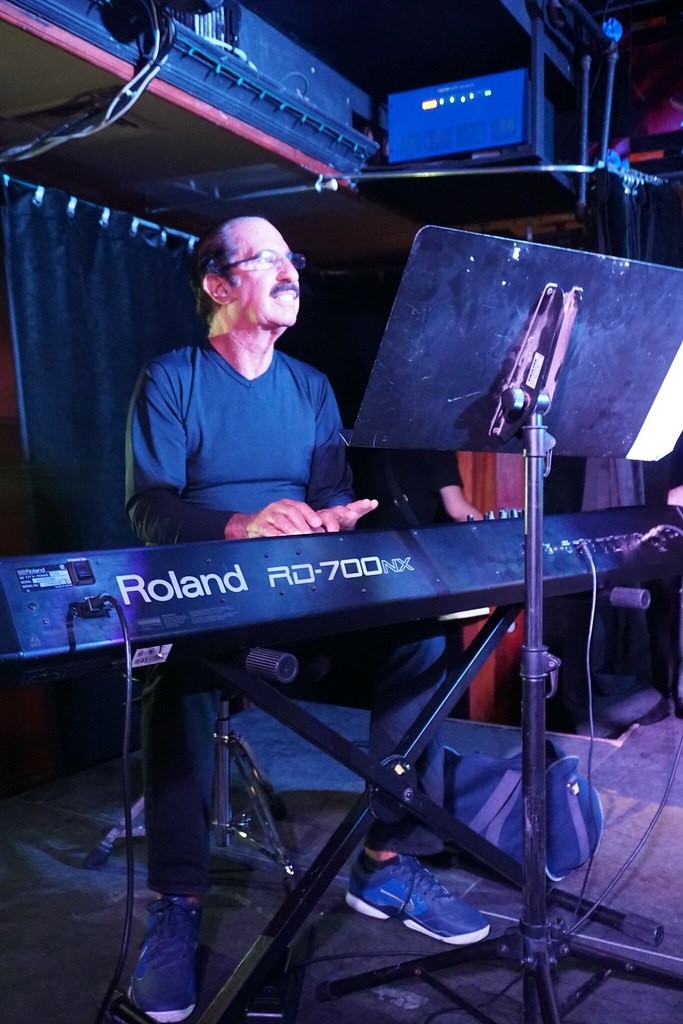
[119,216,491,1024]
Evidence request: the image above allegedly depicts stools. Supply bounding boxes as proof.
[85,652,300,896]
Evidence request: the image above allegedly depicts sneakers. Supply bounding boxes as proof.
[127,895,203,1022]
[347,854,491,945]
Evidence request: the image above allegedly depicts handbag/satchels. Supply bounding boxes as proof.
[442,738,603,879]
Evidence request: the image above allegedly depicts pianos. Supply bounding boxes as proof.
[1,503,683,661]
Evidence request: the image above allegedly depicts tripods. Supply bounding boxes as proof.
[315,223,683,1024]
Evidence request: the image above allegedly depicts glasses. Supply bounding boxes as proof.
[212,250,307,271]
